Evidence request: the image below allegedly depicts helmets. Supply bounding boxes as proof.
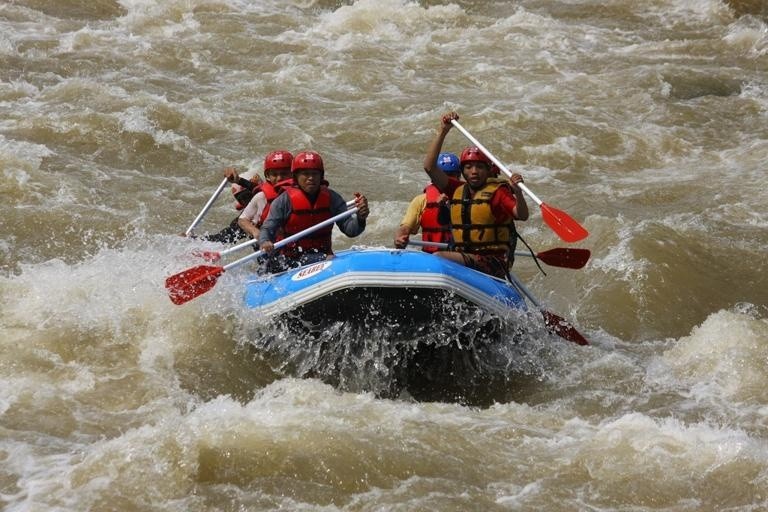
[264,150,294,181]
[290,151,324,183]
[459,146,495,172]
[231,169,263,205]
[436,153,461,177]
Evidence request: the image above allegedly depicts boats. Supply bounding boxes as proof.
[239,249,541,395]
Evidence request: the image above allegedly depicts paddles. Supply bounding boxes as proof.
[443,115,590,242]
[509,273,588,345]
[165,196,362,305]
[401,239,590,270]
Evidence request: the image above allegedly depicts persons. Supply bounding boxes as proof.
[395,112,529,283]
[179,151,368,278]
